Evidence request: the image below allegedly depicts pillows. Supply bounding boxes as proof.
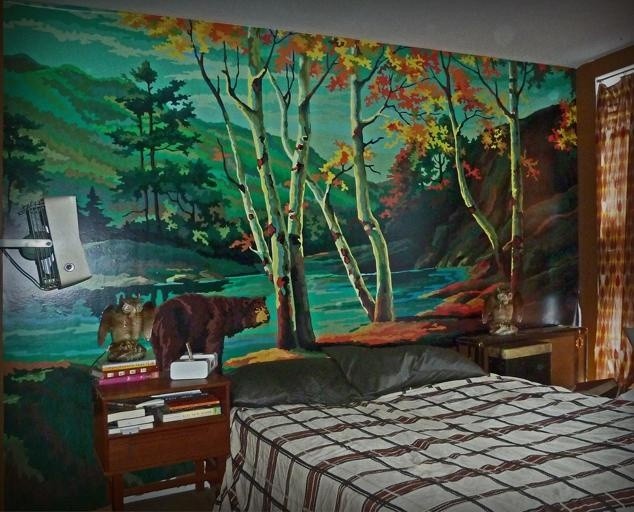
[324,341,489,402]
[225,357,361,407]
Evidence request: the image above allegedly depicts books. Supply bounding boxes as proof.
[92,358,160,386]
[107,390,222,435]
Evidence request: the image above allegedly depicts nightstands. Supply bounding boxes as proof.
[483,324,589,384]
[93,370,231,512]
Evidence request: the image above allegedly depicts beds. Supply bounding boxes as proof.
[212,341,634,512]
